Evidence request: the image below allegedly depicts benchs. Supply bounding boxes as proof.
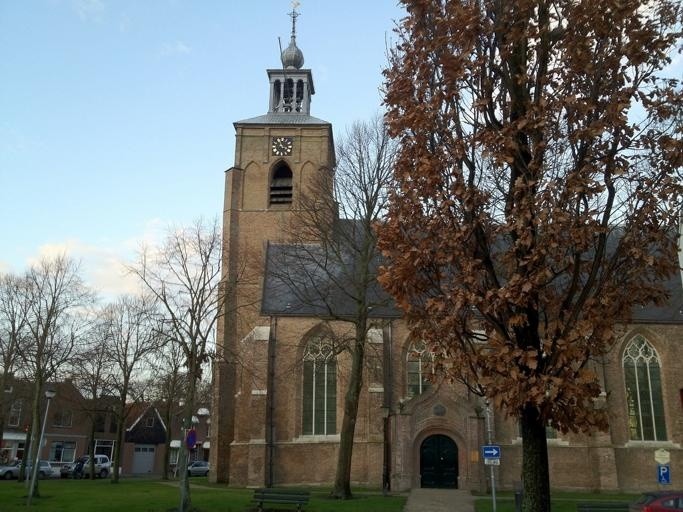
[250,487,310,512]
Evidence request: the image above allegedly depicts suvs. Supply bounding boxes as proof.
[59,454,111,480]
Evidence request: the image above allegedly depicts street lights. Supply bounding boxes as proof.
[24,389,56,505]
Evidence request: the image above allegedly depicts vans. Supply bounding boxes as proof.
[0,459,53,480]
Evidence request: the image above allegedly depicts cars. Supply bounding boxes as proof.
[173,460,209,476]
[629,492,682,511]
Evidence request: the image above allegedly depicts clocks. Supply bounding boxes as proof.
[269,135,295,155]
